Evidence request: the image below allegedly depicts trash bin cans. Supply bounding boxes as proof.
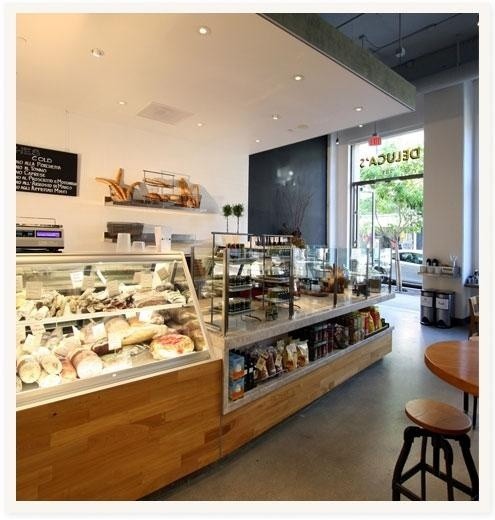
[420,289,456,329]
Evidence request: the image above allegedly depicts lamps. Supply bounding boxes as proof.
[336,130,340,144]
[369,121,381,146]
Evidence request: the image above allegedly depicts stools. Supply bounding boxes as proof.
[392,399,479,501]
[468,295,479,340]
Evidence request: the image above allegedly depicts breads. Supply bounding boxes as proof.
[95,167,201,208]
[16,279,204,392]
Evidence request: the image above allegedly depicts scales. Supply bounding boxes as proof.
[16,223,65,252]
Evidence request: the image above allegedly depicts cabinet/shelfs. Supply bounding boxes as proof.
[16,248,214,411]
[200,292,395,415]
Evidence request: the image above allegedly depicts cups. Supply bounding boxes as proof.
[154,224,160,249]
[117,232,130,253]
[131,242,146,253]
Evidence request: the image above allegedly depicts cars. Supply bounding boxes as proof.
[374,250,423,287]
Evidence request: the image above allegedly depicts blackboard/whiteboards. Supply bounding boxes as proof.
[16,144,77,196]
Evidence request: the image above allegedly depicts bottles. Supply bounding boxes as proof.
[227,243,245,257]
[230,275,251,287]
[229,298,251,311]
[272,303,279,320]
[266,300,273,321]
[159,220,172,251]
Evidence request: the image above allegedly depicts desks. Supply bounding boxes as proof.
[425,340,479,430]
